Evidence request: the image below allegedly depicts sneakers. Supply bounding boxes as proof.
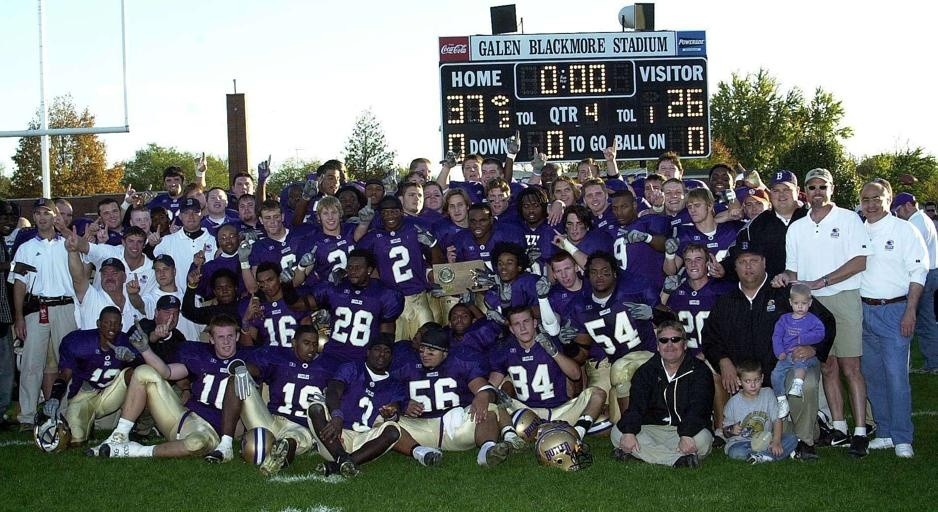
[315,460,361,479]
[1,414,34,435]
[894,443,914,458]
[611,447,631,462]
[84,430,141,460]
[746,452,766,465]
[787,381,803,397]
[910,362,938,376]
[474,433,531,465]
[869,437,894,449]
[789,410,876,461]
[205,445,234,462]
[778,399,790,418]
[413,447,442,465]
[675,454,698,471]
[714,435,725,449]
[261,438,292,477]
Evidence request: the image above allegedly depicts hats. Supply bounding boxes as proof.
[98,255,180,310]
[179,197,201,212]
[891,193,916,211]
[804,167,833,184]
[421,330,450,351]
[0,199,22,215]
[768,169,797,186]
[727,237,779,261]
[32,198,55,212]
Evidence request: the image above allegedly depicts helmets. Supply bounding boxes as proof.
[586,405,615,440]
[533,421,592,471]
[240,428,275,464]
[310,317,331,353]
[33,410,72,456]
[510,408,542,441]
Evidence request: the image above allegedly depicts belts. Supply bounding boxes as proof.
[860,296,908,304]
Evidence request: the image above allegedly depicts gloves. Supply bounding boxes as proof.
[303,175,326,198]
[528,245,579,357]
[357,197,375,223]
[532,147,548,174]
[441,150,464,168]
[718,164,767,206]
[279,244,320,286]
[622,230,680,321]
[259,156,274,179]
[507,133,521,155]
[43,398,60,424]
[496,390,514,410]
[431,282,512,325]
[415,223,434,245]
[107,320,150,362]
[309,308,331,323]
[383,167,399,195]
[239,232,259,264]
[234,366,259,401]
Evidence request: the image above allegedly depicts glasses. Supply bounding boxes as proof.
[657,337,684,343]
[805,186,828,192]
